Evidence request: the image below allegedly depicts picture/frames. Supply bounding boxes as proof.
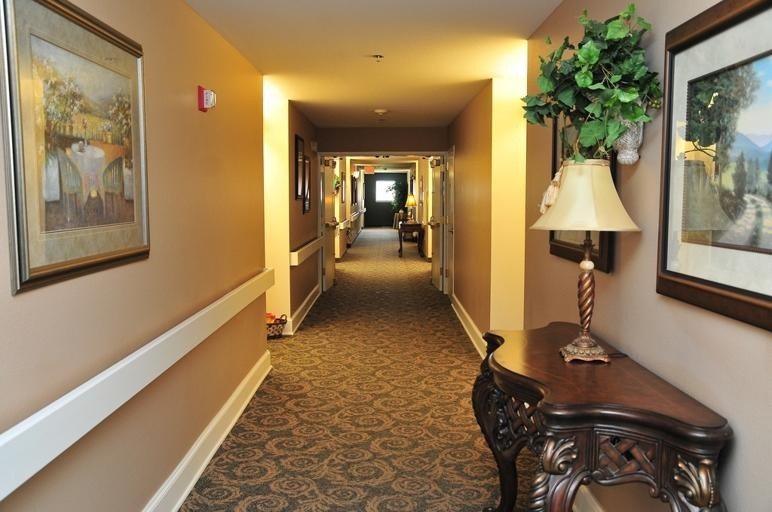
[653,0,771,330]
[3,2,150,297]
[293,133,311,213]
[547,101,618,274]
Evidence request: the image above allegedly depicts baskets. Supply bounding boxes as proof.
[267,314,287,338]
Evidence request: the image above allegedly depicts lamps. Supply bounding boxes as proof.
[405,195,417,224]
[529,157,640,367]
[475,320,733,512]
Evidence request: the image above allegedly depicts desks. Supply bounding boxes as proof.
[398,221,425,258]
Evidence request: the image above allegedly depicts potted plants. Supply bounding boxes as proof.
[520,1,665,167]
